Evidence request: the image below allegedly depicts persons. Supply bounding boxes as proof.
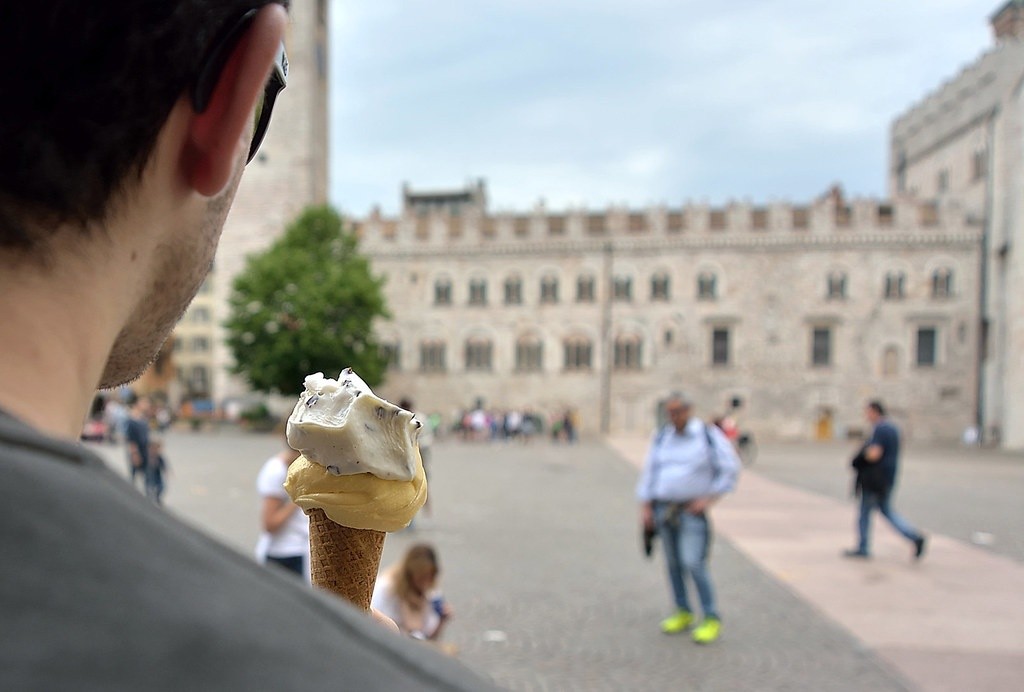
[636,400,742,643]
[847,403,925,557]
[369,544,451,641]
[715,397,750,454]
[0,0,493,692]
[431,397,578,443]
[90,390,215,506]
[256,446,309,578]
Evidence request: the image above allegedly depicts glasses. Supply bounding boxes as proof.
[245,8,289,164]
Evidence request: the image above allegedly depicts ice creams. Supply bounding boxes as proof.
[282,366,429,612]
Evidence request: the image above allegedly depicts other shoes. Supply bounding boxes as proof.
[661,611,695,634]
[845,546,870,556]
[691,616,722,645]
[916,537,925,556]
